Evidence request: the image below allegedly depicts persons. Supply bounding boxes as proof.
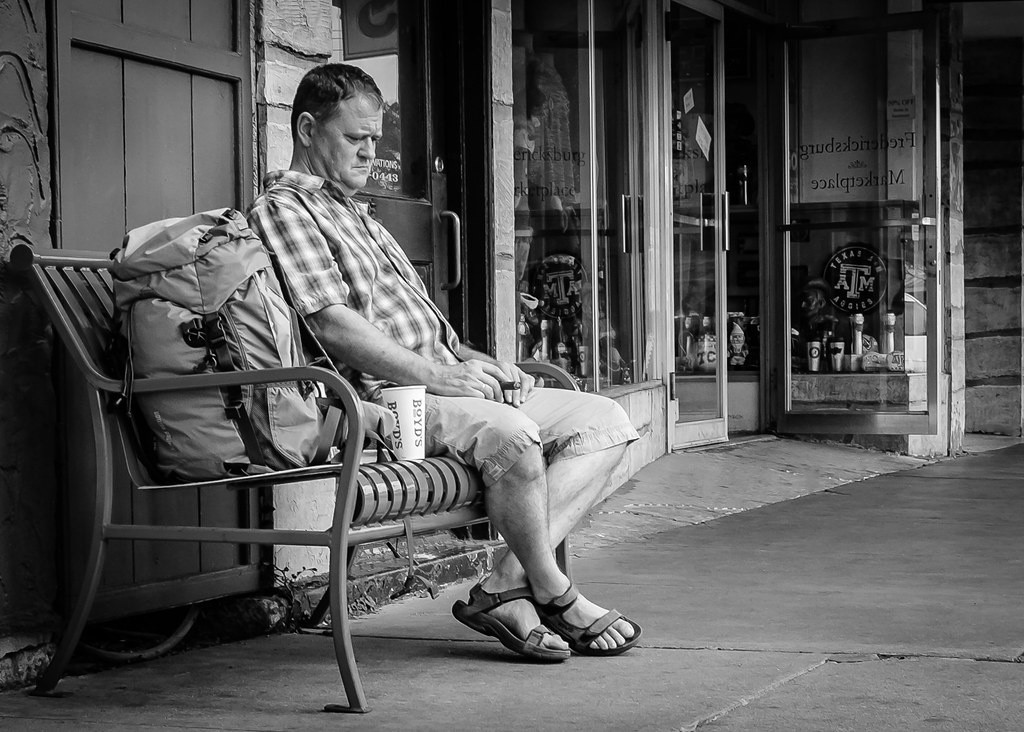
[242,63,643,663]
[798,285,839,341]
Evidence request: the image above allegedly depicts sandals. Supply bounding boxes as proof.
[539,583,642,656]
[452,583,571,659]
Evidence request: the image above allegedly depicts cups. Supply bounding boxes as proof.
[830,337,844,372]
[381,385,426,461]
[578,344,592,376]
[807,337,821,372]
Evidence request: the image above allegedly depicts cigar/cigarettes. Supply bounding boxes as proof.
[500,382,522,390]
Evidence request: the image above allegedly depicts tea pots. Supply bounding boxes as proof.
[678,330,717,372]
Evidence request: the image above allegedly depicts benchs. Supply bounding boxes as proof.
[9,244,580,713]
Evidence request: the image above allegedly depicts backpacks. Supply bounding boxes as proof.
[109,207,399,480]
